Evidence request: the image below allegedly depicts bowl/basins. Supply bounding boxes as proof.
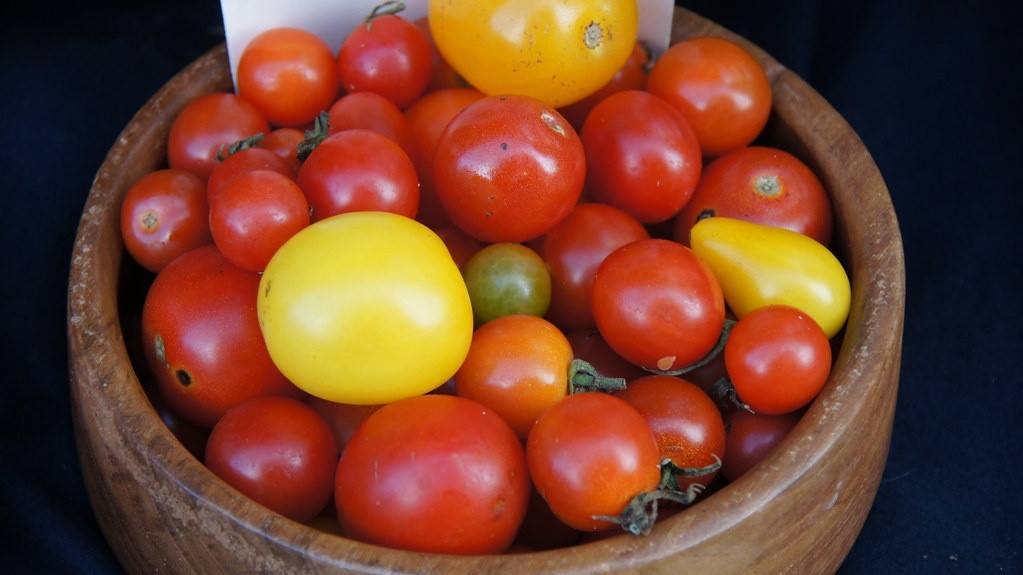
[63,4,909,574]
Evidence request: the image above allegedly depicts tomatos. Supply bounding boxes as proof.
[121,0,852,556]
